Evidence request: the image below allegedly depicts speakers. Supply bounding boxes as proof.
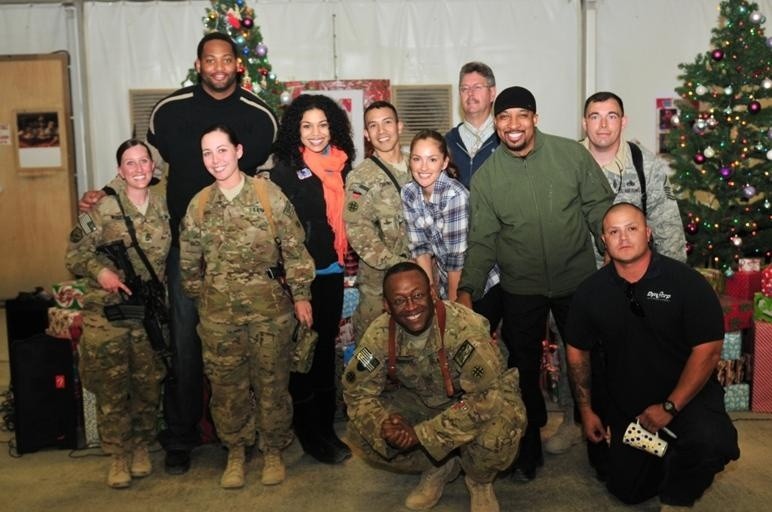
[4,298,87,455]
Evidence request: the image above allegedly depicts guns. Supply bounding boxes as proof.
[97,239,176,382]
[268,266,288,288]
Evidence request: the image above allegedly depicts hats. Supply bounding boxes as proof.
[494,87,536,117]
[290,320,320,375]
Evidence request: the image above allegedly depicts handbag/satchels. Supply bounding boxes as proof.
[146,279,175,325]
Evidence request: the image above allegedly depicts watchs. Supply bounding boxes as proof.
[662,396,679,416]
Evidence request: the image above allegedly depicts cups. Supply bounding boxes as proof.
[620,417,672,459]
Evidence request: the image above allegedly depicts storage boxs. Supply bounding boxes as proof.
[695,258,772,411]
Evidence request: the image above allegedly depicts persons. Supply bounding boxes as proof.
[339,262,529,511]
[561,203,742,510]
[65,140,172,488]
[140,32,687,464]
[178,131,317,490]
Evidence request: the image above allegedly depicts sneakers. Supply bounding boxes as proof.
[107,454,132,488]
[302,433,352,465]
[510,433,542,482]
[220,445,246,488]
[405,456,462,510]
[464,474,500,512]
[261,445,287,485]
[544,422,587,454]
[129,446,152,477]
[165,449,190,474]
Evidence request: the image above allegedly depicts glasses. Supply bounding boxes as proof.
[625,282,646,318]
[459,83,495,93]
